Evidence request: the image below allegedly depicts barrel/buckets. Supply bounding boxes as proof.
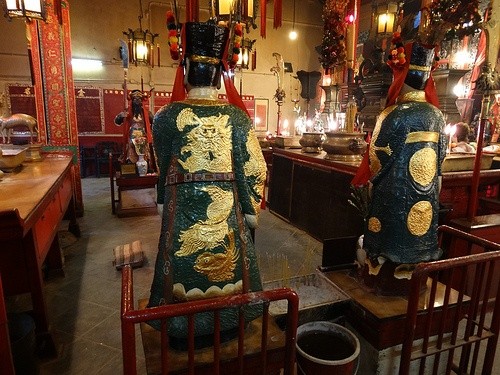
[295,321,361,375]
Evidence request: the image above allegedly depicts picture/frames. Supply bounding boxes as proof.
[255,98,269,132]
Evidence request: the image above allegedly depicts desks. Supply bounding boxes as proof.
[0,151,83,358]
[268,147,500,244]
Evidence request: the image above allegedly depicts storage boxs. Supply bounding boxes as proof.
[8,271,69,333]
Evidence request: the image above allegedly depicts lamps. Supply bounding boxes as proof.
[123,0,160,92]
[209,0,282,38]
[236,38,256,100]
[0,0,47,85]
[372,0,397,52]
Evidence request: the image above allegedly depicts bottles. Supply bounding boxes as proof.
[137,154,147,177]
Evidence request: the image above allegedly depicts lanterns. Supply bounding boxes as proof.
[210,0,283,70]
[122,26,161,68]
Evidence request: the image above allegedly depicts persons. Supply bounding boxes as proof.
[451,122,476,152]
[364,43,447,295]
[144,21,268,351]
[115,90,154,163]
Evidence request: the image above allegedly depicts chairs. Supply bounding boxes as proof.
[122,265,298,375]
[317,225,500,375]
[96,141,120,178]
[108,152,159,218]
